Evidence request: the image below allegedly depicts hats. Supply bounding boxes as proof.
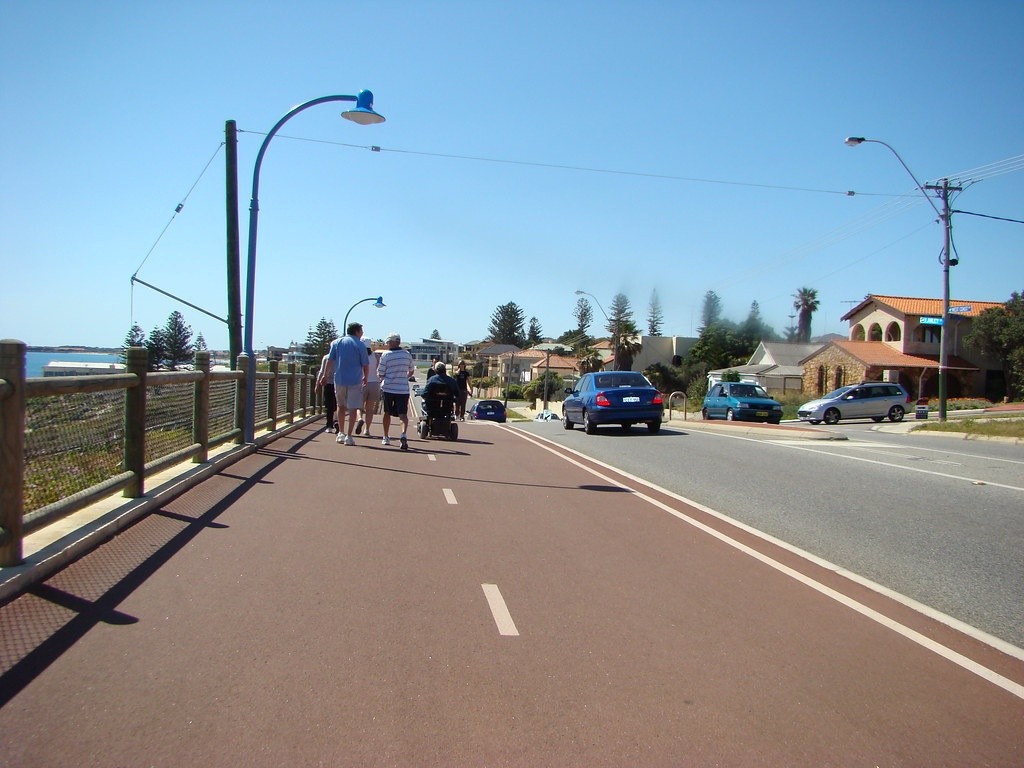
[385,333,400,343]
[361,337,371,348]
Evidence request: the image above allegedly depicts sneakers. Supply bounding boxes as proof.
[336,435,345,443]
[400,434,408,449]
[382,438,390,444]
[344,438,355,445]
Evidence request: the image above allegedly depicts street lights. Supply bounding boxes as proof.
[241,88,387,446]
[844,137,951,426]
[343,295,387,338]
[574,290,620,371]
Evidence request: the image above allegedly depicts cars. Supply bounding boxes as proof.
[701,382,784,424]
[559,370,665,435]
[414,388,421,397]
[465,399,507,423]
[533,411,560,422]
[412,384,420,391]
[408,376,415,382]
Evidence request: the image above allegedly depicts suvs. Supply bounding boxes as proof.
[796,381,913,426]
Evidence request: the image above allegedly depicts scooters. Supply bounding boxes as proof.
[415,392,459,442]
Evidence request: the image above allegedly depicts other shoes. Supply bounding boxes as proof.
[333,423,339,434]
[460,418,464,421]
[364,431,370,437]
[355,419,364,434]
[325,428,332,432]
[455,415,459,419]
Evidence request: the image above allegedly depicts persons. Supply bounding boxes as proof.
[320,323,369,446]
[355,338,381,436]
[377,332,414,450]
[455,359,473,421]
[427,357,439,380]
[424,362,462,402]
[314,353,339,435]
